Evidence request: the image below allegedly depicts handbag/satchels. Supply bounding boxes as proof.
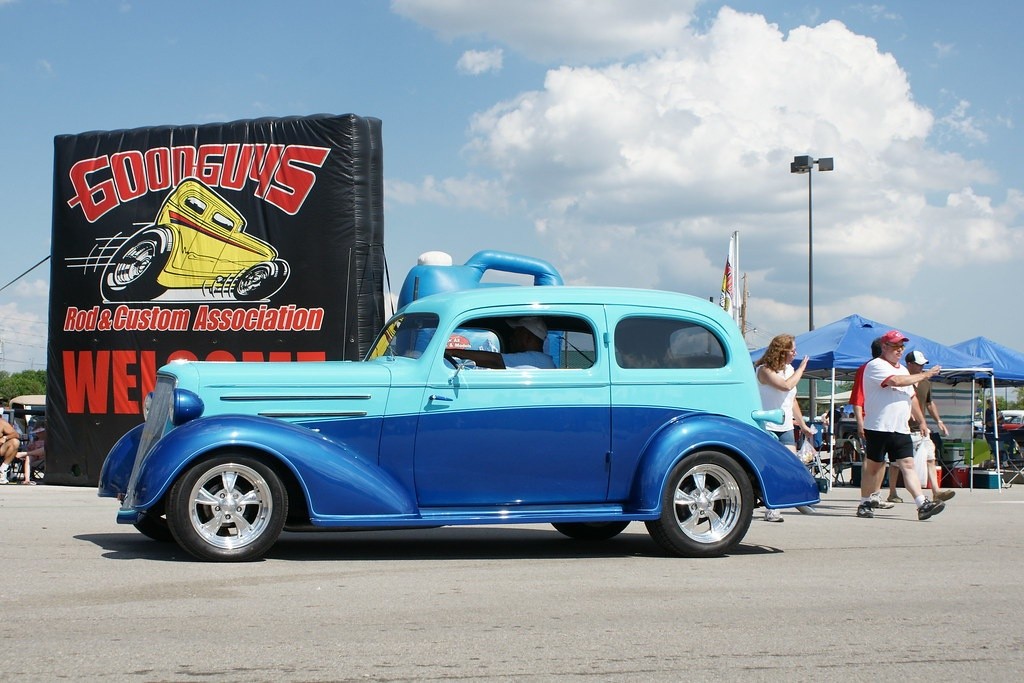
[811,455,828,492]
[795,424,818,463]
[914,436,927,487]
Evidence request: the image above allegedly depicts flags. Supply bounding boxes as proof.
[719,235,733,319]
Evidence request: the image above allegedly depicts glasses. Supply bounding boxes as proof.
[884,342,905,350]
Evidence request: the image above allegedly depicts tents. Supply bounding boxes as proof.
[749,315,1001,495]
[945,336,1024,492]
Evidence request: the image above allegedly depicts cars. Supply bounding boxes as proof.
[98,286,821,562]
[975,409,1023,431]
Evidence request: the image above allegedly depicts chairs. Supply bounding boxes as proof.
[803,434,854,487]
[11,458,44,485]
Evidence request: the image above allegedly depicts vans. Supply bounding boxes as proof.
[9,395,47,448]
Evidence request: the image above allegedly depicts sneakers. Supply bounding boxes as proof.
[933,490,956,502]
[857,502,874,517]
[871,495,894,508]
[764,508,784,522]
[888,495,902,502]
[0,469,10,484]
[917,496,945,520]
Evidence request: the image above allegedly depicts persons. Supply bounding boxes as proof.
[886,350,955,503]
[825,404,841,441]
[499,317,559,369]
[985,396,1008,468]
[839,406,847,418]
[849,413,854,418]
[0,408,20,485]
[15,420,45,485]
[856,330,946,520]
[849,336,885,502]
[821,409,829,433]
[755,334,818,522]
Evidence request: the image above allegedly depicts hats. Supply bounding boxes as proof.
[905,351,929,366]
[505,316,548,341]
[881,331,909,344]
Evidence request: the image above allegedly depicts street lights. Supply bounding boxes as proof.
[791,155,834,422]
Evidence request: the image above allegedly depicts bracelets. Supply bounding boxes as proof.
[936,421,943,424]
[3,437,7,441]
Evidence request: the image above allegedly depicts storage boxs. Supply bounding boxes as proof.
[851,440,1003,489]
